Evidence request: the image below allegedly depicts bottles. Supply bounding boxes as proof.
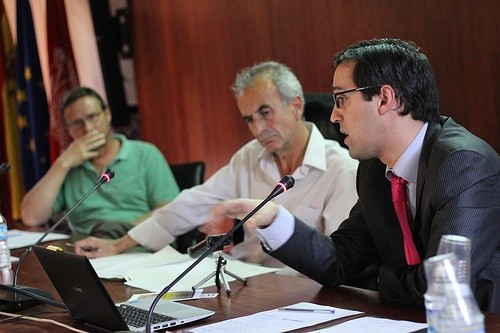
[0,213,15,286]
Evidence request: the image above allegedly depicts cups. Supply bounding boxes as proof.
[437,234,471,284]
[424,252,486,333]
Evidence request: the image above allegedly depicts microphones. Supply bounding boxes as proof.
[0,169,116,313]
[146,174,295,333]
[0,163,10,173]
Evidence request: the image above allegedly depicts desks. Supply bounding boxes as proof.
[0,239,500,333]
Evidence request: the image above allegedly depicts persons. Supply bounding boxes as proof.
[20,87,181,242]
[211,37,500,313]
[75,62,360,279]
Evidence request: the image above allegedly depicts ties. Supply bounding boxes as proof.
[390,175,422,265]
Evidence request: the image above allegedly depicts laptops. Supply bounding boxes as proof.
[30,245,216,333]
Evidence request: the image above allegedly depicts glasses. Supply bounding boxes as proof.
[330,83,383,108]
[66,109,105,131]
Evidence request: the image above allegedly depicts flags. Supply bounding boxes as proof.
[0,0,141,192]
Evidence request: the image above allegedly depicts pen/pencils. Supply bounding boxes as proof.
[279,306,336,313]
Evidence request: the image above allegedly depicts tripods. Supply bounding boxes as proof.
[192,246,248,294]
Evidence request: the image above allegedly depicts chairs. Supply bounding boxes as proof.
[169,161,204,250]
